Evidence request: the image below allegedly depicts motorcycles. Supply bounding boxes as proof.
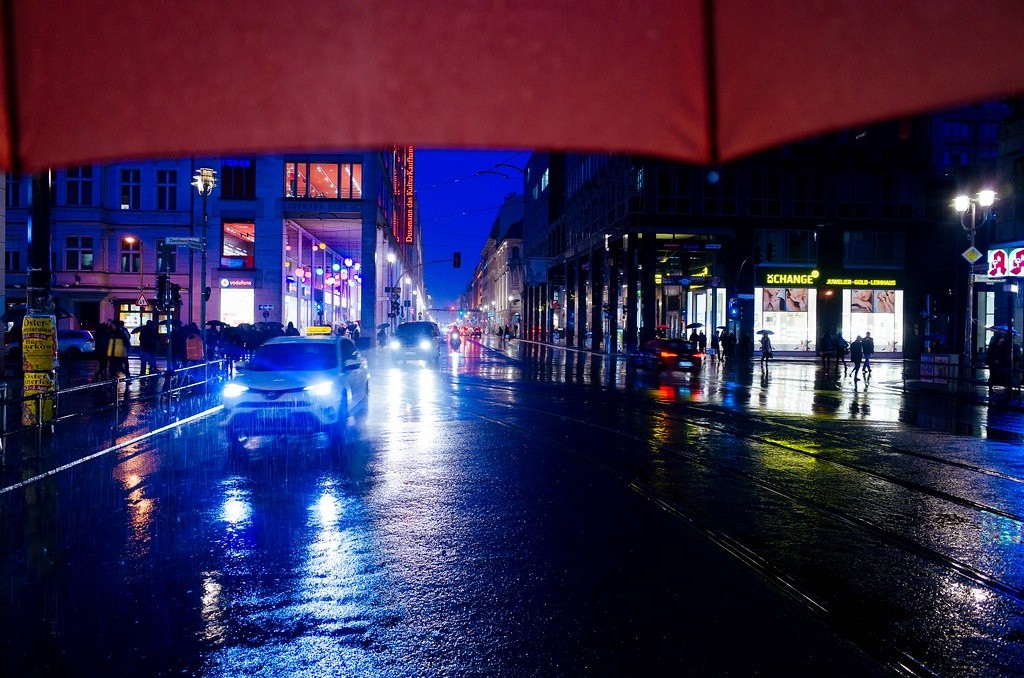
[449,332,463,351]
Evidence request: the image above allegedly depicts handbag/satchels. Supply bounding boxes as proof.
[769,350,773,358]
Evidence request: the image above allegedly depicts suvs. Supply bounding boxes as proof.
[219,325,369,454]
[629,339,701,376]
[4,328,96,363]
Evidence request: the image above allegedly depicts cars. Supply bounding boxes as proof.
[459,327,481,338]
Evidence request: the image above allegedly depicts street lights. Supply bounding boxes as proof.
[191,167,220,362]
[949,191,1001,386]
[127,236,147,327]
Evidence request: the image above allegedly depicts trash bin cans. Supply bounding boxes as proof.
[948,349,965,393]
[186,334,203,360]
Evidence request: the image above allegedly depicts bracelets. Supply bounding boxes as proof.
[799,304,806,307]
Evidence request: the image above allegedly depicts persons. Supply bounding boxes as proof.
[377,329,388,347]
[689,329,737,362]
[95,319,300,380]
[853,290,895,313]
[763,288,807,312]
[761,334,772,366]
[984,333,1002,392]
[450,326,459,333]
[820,331,874,380]
[497,324,517,341]
[323,321,360,340]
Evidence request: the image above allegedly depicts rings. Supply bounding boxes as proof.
[774,300,777,301]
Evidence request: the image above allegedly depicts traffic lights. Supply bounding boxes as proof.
[451,306,455,310]
[388,314,395,317]
[171,284,183,307]
[391,301,394,310]
[394,303,398,308]
[408,300,411,307]
[459,311,463,316]
[392,287,402,300]
[404,300,407,307]
[385,287,390,292]
[397,303,401,315]
[317,303,323,315]
[155,276,167,312]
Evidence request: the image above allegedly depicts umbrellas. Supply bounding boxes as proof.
[377,324,390,329]
[0,305,73,322]
[131,326,145,334]
[685,323,703,328]
[717,326,730,330]
[159,319,183,325]
[757,330,775,341]
[206,320,225,326]
[987,325,1021,338]
[0,0,1023,187]
